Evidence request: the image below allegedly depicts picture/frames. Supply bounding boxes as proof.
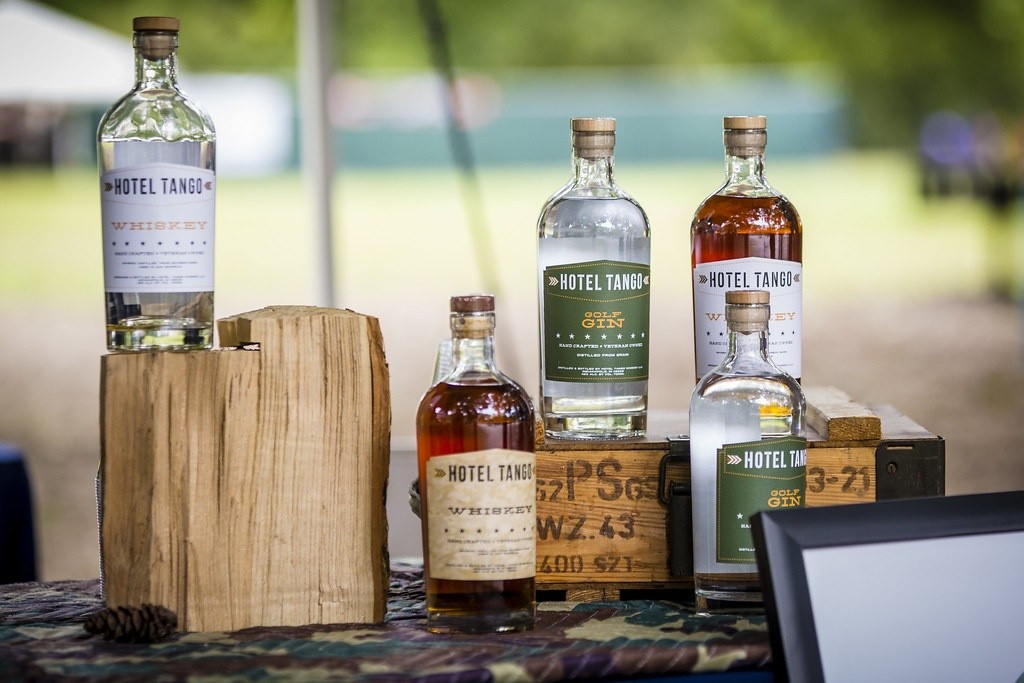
[749,490,1024,683]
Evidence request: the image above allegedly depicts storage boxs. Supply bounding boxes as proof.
[533,405,946,609]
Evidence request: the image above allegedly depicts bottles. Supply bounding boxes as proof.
[415,295,538,636]
[96,17,217,353]
[687,288,809,616]
[690,116,803,396]
[536,116,651,442]
